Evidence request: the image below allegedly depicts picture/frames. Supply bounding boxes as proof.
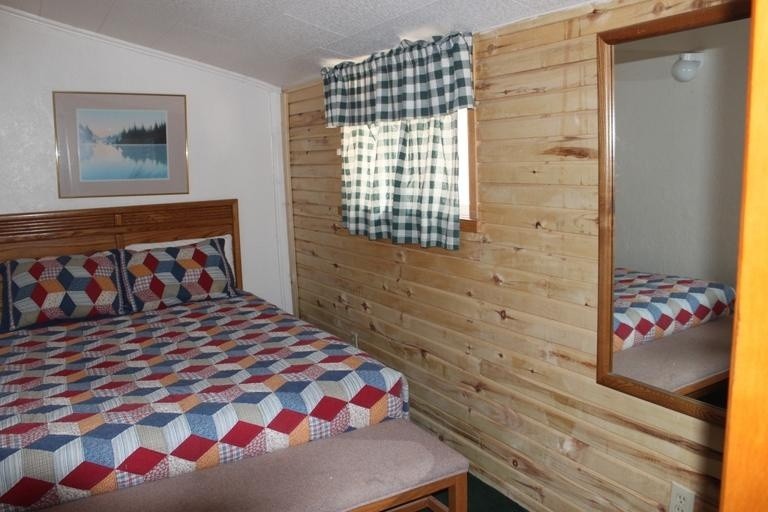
[53,91,191,198]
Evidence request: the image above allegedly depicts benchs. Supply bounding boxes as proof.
[24,415,468,512]
[611,310,732,396]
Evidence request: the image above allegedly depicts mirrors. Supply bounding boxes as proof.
[595,2,753,427]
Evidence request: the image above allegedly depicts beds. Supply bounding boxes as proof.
[611,265,735,354]
[0,199,411,511]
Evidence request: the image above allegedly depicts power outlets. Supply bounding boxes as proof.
[666,480,697,508]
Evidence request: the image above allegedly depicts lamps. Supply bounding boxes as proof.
[670,50,705,83]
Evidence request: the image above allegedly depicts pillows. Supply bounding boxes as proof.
[128,234,236,277]
[1,250,134,333]
[120,239,236,312]
